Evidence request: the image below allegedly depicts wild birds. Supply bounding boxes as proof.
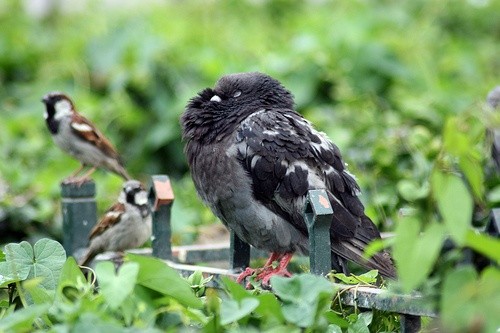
[41,93,129,190]
[181,71,398,292]
[79,180,153,272]
